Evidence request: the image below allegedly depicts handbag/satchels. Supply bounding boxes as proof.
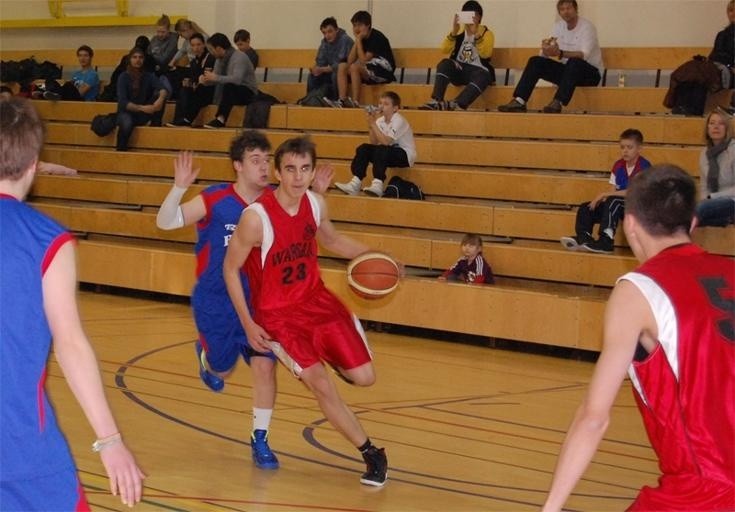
[297,84,333,107]
[382,176,425,201]
[92,113,116,136]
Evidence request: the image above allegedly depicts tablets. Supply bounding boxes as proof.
[454,11,477,26]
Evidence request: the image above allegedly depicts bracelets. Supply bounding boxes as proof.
[91,432,123,453]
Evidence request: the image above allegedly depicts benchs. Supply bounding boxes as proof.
[0,47,735,353]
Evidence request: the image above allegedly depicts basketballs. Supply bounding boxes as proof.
[347,250,400,301]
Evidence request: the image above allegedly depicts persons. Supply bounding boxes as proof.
[334,90,417,197]
[694,107,735,227]
[436,232,495,286]
[667,0,735,117]
[0,96,148,512]
[105,13,258,152]
[296,18,356,106]
[540,161,735,512]
[156,130,335,472]
[417,1,496,110]
[0,85,14,104]
[560,126,653,254]
[223,132,389,486]
[31,45,100,102]
[498,0,605,112]
[322,11,396,108]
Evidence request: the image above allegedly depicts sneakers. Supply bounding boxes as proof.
[196,339,224,392]
[363,178,384,197]
[323,95,357,107]
[166,119,192,128]
[204,119,225,128]
[359,445,389,487]
[334,175,362,195]
[715,104,735,117]
[251,429,279,470]
[420,100,455,110]
[32,91,60,100]
[560,231,615,253]
[500,100,562,113]
